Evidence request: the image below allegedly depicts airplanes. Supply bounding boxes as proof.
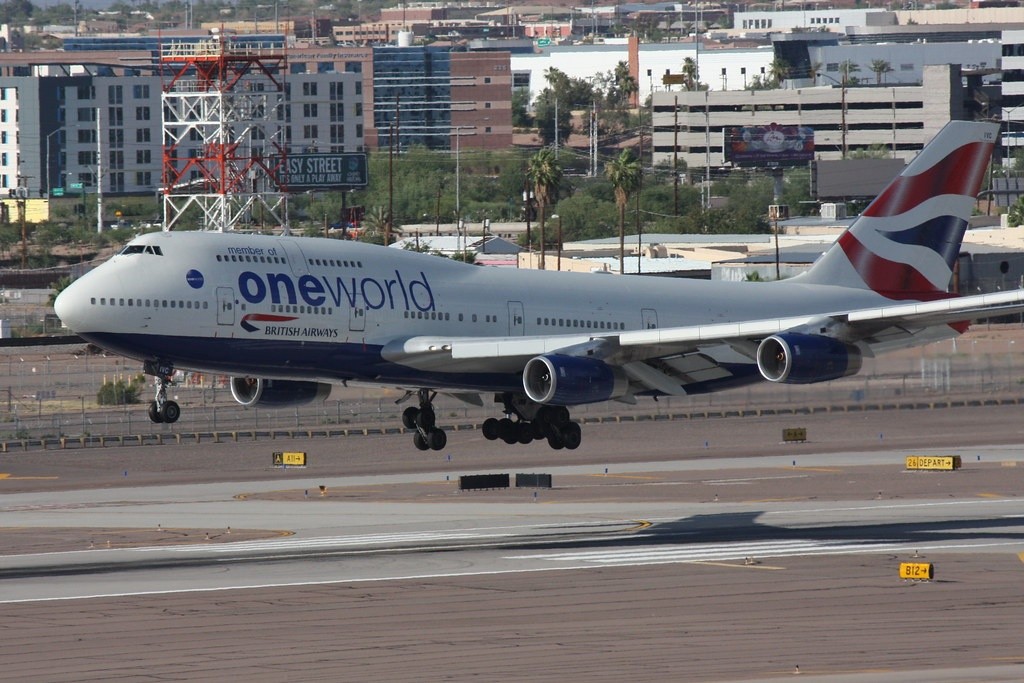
[53,121,1024,451]
